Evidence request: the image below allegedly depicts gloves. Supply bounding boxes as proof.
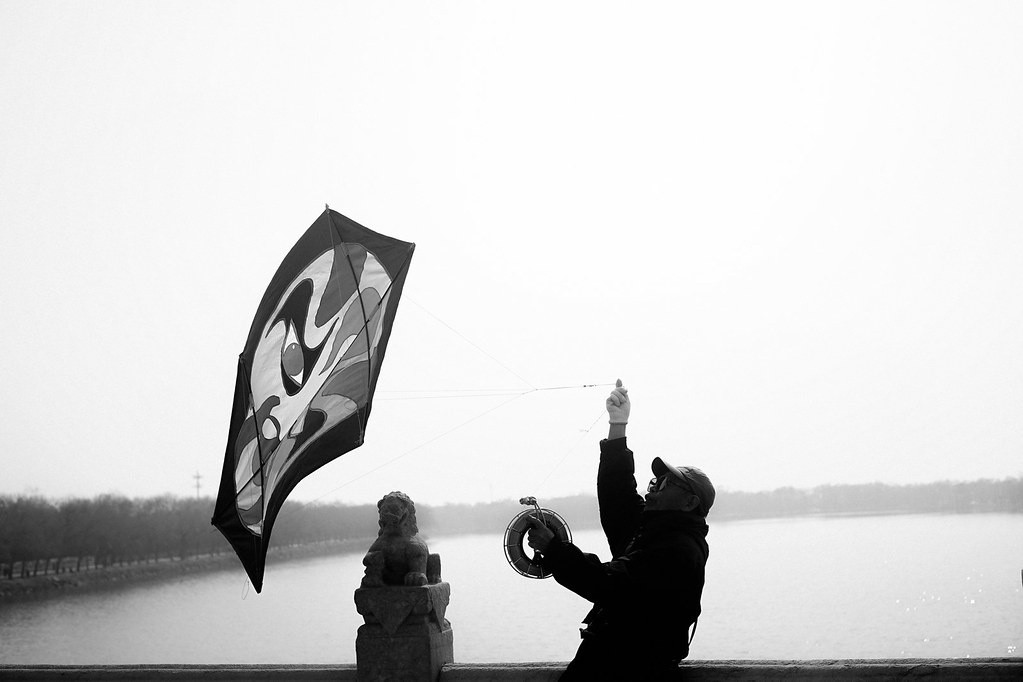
[605,378,630,424]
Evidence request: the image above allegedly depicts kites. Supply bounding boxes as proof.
[210,208,416,593]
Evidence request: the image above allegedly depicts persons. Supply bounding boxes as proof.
[527,380,718,682]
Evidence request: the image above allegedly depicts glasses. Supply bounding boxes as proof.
[647,476,696,494]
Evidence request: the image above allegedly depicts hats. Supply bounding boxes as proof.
[651,458,715,513]
